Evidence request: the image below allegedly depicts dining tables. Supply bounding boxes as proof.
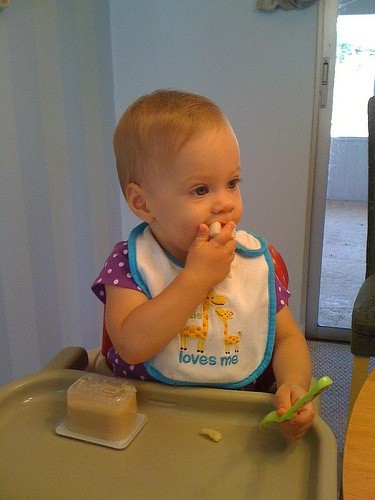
[0,347,339,500]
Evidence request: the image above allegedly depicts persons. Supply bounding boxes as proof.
[91,89,315,442]
